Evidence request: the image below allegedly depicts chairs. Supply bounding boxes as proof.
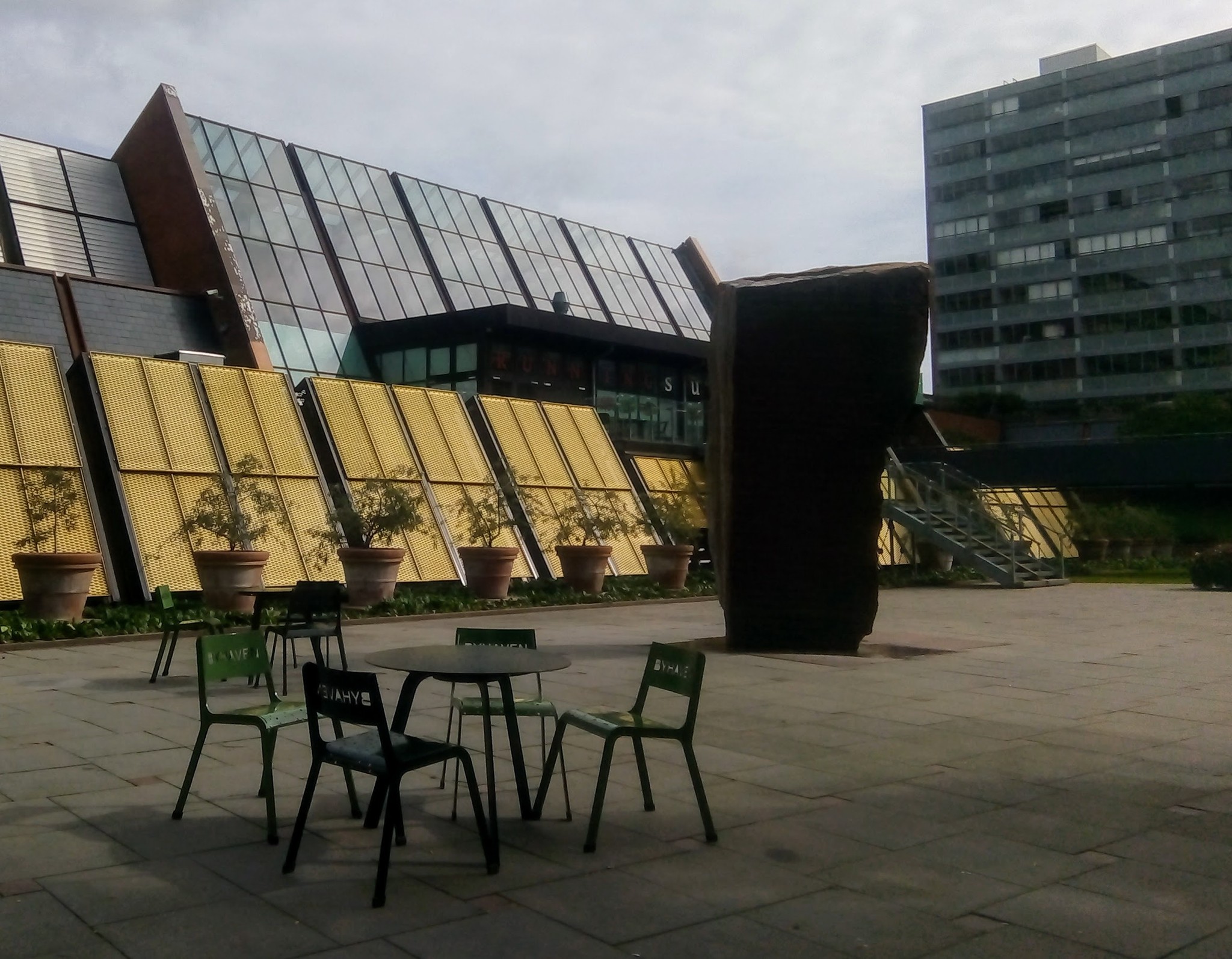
[253,580,348,696]
[149,585,228,685]
[283,662,498,908]
[171,630,363,845]
[439,629,573,826]
[532,641,718,851]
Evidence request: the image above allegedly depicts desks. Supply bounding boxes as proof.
[364,647,572,836]
[236,588,294,684]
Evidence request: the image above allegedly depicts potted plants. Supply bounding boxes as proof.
[635,468,701,593]
[145,452,295,611]
[438,456,546,600]
[304,465,437,604]
[546,478,634,593]
[10,461,102,623]
